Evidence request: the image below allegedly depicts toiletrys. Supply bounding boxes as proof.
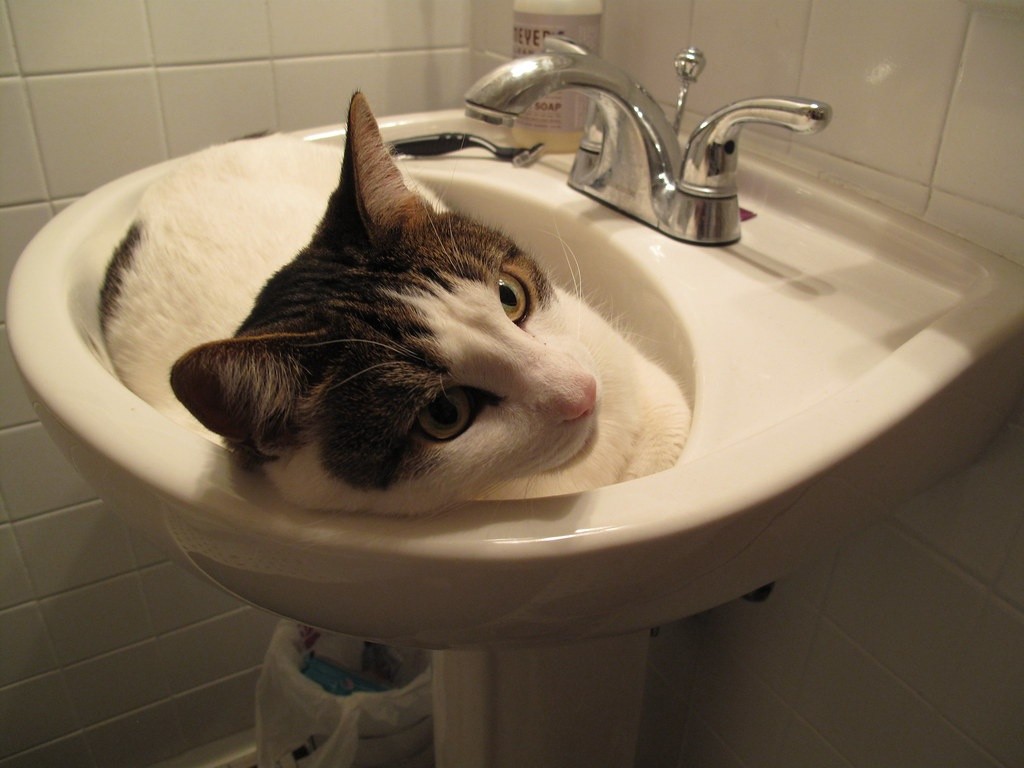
[510,2,608,156]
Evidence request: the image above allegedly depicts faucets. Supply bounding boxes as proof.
[460,34,833,247]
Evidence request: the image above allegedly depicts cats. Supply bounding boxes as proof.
[100,88,689,510]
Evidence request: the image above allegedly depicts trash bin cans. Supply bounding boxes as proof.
[266,618,437,768]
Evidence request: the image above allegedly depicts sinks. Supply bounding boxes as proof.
[2,99,1024,649]
